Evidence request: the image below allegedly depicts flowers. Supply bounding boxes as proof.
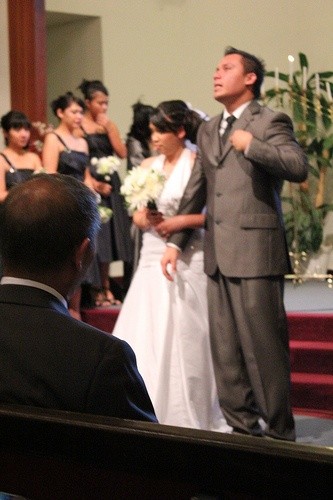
[120,166,167,216]
[95,155,122,176]
[99,205,113,224]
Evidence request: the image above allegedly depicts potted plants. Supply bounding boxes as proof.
[262,53,333,275]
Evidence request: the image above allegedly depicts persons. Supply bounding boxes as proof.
[110,100,233,434]
[162,47,309,442]
[0,172,160,423]
[81,78,135,306]
[42,92,112,321]
[0,110,44,203]
